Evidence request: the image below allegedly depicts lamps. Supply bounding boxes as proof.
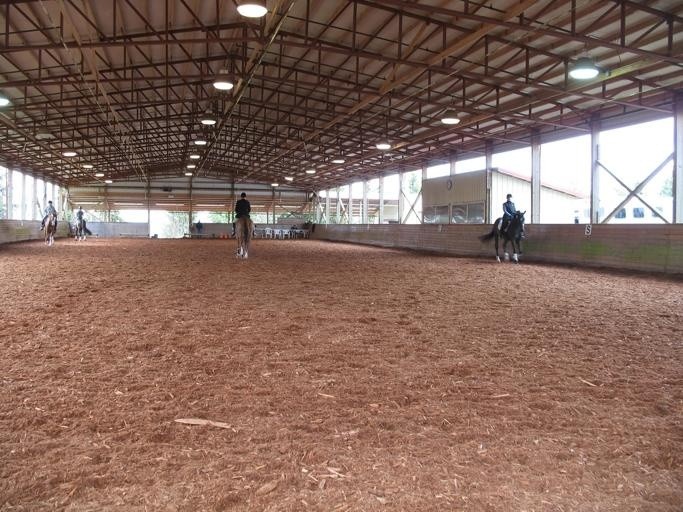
[569,41,601,80]
[440,106,460,123]
[213,57,234,91]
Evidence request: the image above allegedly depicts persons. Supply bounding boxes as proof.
[500,194,525,238]
[196,220,203,239]
[39,201,57,233]
[75,206,83,225]
[231,192,257,236]
[291,225,297,229]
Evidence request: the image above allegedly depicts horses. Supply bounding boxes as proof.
[232,216,254,259]
[41,211,60,245]
[477,211,528,264]
[71,215,93,242]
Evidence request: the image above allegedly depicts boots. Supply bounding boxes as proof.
[253,230,256,236]
[231,223,235,236]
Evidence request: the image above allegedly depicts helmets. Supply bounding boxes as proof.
[507,194,512,199]
[241,193,246,197]
